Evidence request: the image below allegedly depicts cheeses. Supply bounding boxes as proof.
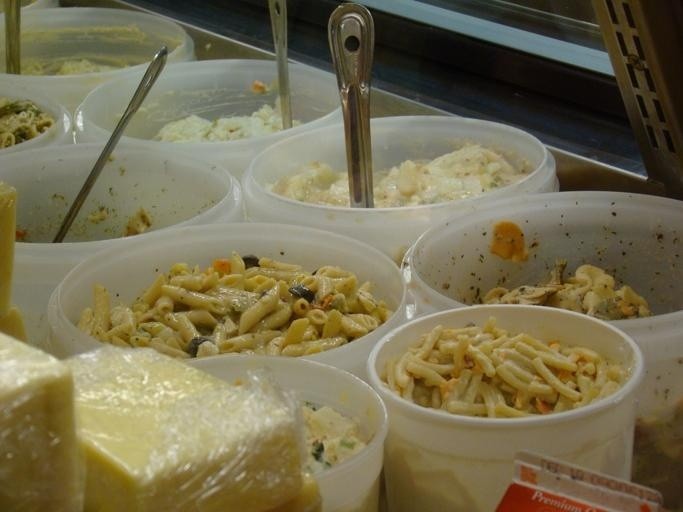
[0,180,325,512]
